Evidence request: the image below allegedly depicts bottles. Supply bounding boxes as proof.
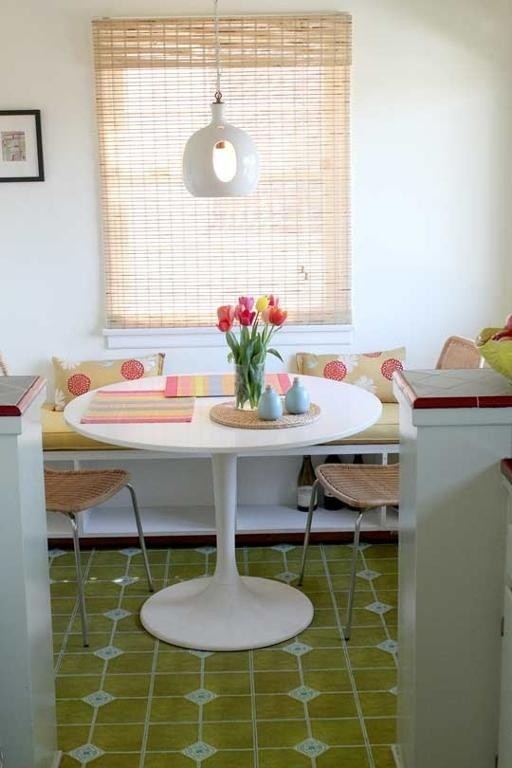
[347,454,364,511]
[285,377,310,414]
[258,384,282,421]
[296,455,317,512]
[323,455,343,510]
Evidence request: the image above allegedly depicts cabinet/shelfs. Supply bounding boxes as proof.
[391,369,511,768]
[44,451,401,547]
[0,376,61,768]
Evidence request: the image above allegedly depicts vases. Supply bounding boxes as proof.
[233,363,265,412]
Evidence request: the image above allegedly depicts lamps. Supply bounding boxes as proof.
[182,0,261,199]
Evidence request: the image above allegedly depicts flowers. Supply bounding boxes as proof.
[214,293,287,408]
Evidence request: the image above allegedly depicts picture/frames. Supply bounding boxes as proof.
[0,109,45,183]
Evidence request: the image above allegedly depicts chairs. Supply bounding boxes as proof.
[297,336,484,641]
[0,362,154,649]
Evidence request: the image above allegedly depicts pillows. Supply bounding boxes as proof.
[53,352,164,411]
[296,348,406,404]
